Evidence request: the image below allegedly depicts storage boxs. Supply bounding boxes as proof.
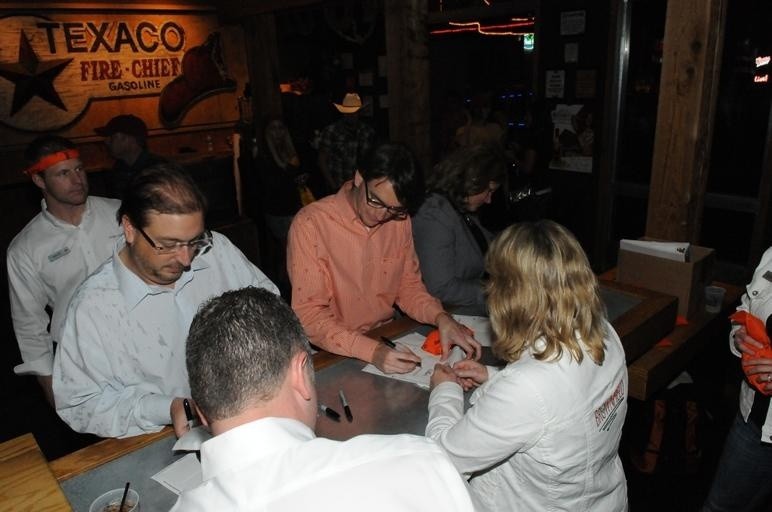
[616,235,714,320]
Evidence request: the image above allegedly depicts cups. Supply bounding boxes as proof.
[87,487,147,511]
[703,285,725,313]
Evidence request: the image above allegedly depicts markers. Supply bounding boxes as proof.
[339,389,353,421]
[319,403,340,420]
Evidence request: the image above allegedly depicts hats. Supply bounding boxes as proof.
[91,113,148,141]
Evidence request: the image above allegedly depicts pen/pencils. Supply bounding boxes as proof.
[380,335,422,367]
[182,398,200,465]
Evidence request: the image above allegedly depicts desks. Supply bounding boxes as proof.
[60,277,682,510]
[1,424,71,512]
[592,267,745,477]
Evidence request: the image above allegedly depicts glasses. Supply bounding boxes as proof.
[131,224,214,256]
[364,178,408,221]
